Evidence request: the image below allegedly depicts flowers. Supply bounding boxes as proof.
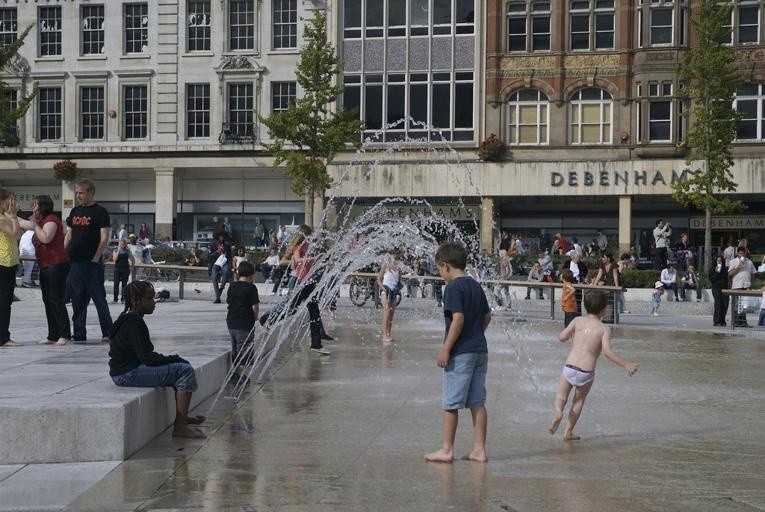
[54,161,78,184]
[476,133,513,162]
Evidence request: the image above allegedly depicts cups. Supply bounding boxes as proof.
[716,264,721,273]
[739,256,745,267]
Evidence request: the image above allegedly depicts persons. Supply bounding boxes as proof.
[547,289,638,441]
[465,219,765,328]
[106,279,207,440]
[423,241,492,463]
[1,177,445,386]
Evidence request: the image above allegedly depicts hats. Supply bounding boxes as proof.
[655,281,664,289]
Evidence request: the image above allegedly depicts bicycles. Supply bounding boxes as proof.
[350,273,402,308]
[138,248,179,284]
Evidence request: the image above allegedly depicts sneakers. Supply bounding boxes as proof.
[21,281,39,289]
[3,334,113,346]
[311,347,331,355]
[230,372,250,385]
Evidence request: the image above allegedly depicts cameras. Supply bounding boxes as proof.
[668,224,672,228]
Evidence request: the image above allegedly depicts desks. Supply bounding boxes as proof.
[346,272,627,325]
[19,255,235,300]
[722,289,762,331]
